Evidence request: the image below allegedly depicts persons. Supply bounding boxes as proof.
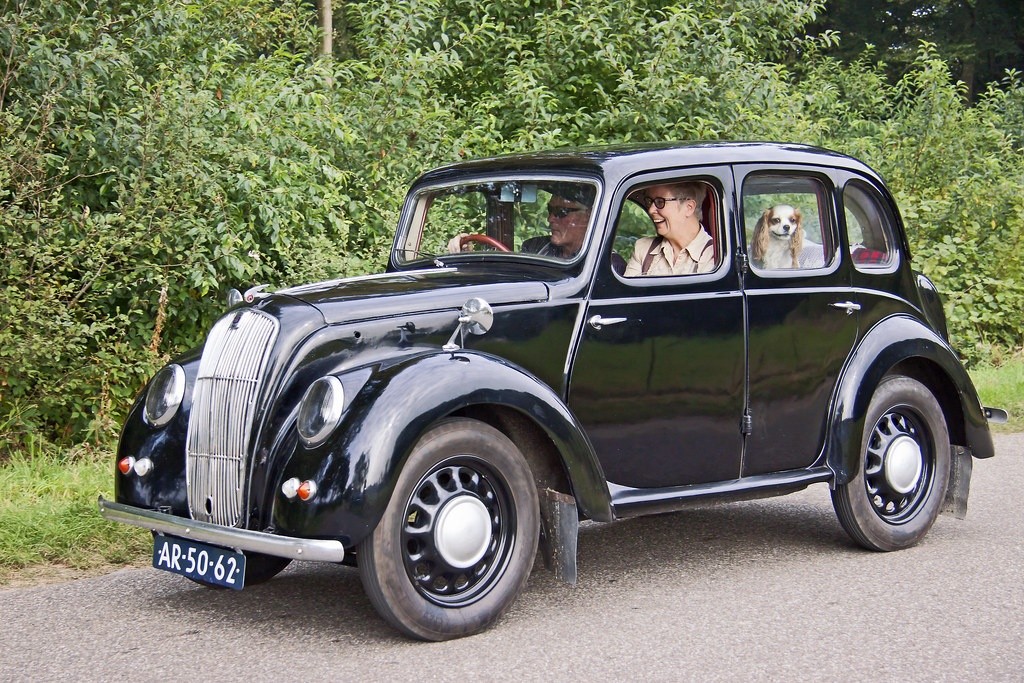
[621,181,715,276]
[446,189,626,275]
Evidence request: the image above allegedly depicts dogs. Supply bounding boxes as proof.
[748,204,803,272]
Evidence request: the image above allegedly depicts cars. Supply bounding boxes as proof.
[98,139,1011,645]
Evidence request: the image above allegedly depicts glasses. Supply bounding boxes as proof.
[644,197,691,209]
[547,202,582,218]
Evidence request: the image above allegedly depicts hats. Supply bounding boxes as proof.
[552,181,596,210]
